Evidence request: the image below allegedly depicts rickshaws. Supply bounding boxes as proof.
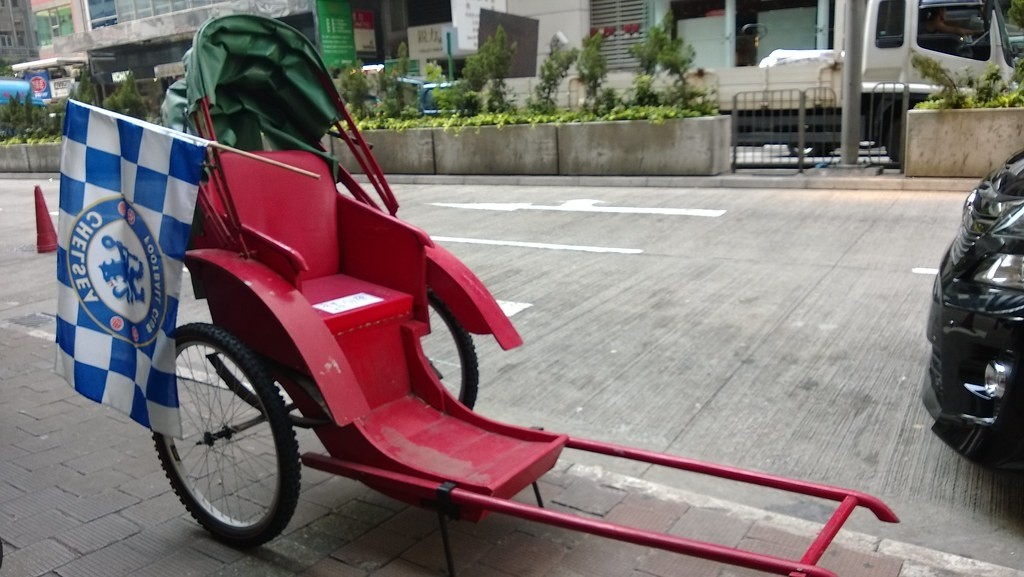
[150,11,901,577]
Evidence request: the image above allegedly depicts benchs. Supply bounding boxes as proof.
[196,146,437,407]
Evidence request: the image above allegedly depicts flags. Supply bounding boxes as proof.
[54,101,206,439]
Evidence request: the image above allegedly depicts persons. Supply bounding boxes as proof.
[920,8,985,53]
[165,75,182,92]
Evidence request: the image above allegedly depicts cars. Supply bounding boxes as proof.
[921,146,1024,473]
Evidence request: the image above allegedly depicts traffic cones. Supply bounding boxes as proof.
[35,186,59,252]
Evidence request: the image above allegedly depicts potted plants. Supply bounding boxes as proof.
[321,11,732,178]
[0,64,150,173]
[906,48,1024,178]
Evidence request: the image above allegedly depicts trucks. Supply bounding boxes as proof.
[487,0,1020,165]
